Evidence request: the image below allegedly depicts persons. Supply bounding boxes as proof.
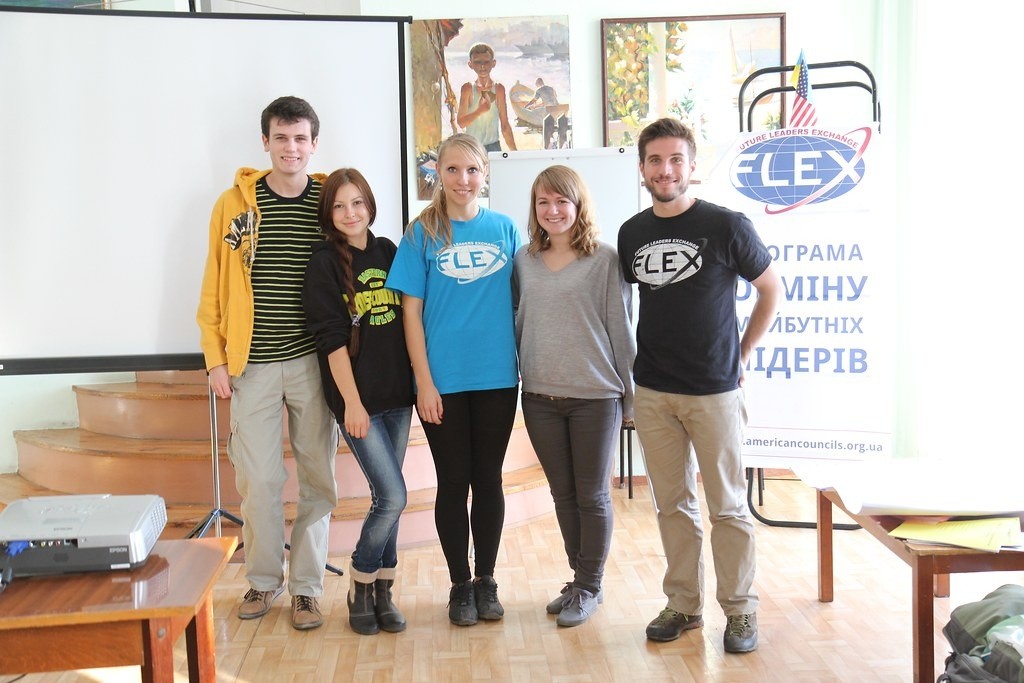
[385,132,522,628]
[194,94,340,631]
[302,168,416,635]
[617,118,781,654]
[509,165,638,631]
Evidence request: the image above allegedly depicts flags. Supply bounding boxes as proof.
[788,50,817,128]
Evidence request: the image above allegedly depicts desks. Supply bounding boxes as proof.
[816,487,1024,683]
[0,535,239,683]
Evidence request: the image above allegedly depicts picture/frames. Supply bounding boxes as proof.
[600,11,787,148]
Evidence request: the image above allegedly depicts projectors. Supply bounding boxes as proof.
[0,494,167,577]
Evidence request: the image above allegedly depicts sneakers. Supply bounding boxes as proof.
[291,595,322,630]
[236,578,287,619]
[723,611,757,653]
[446,581,478,627]
[545,582,603,614]
[645,608,702,642]
[556,586,598,627]
[473,579,504,620]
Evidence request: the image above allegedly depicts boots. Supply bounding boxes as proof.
[347,561,380,635]
[374,567,407,632]
